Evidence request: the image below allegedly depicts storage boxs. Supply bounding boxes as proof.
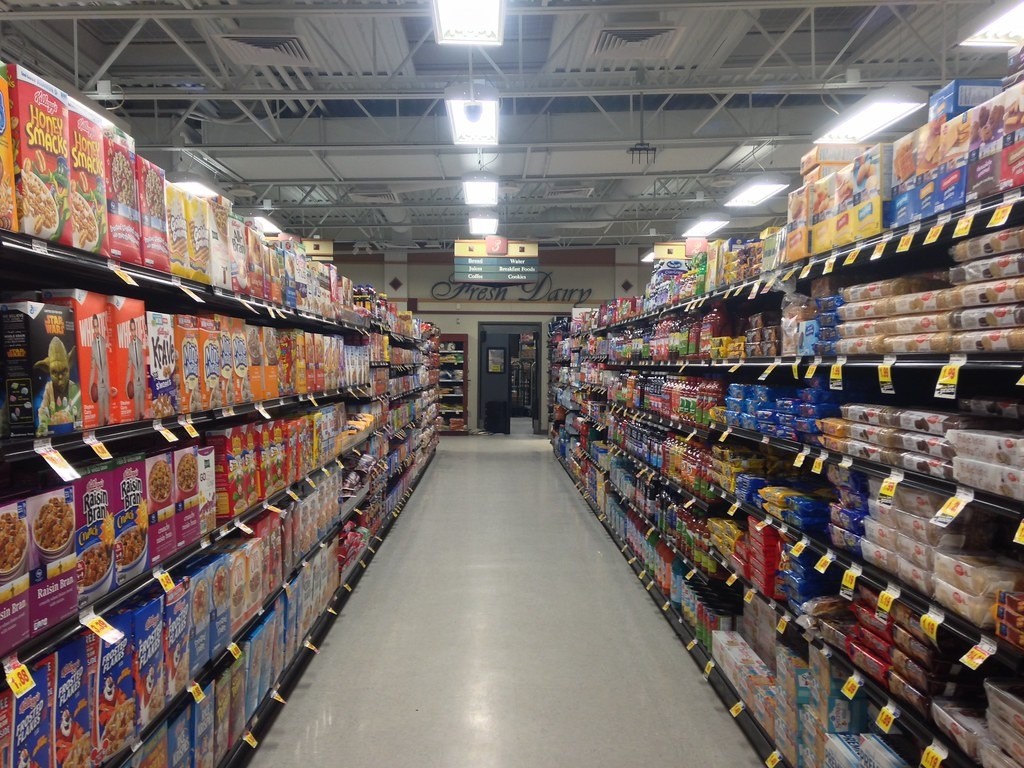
[0,301,83,439]
[707,44,1024,768]
[0,61,389,768]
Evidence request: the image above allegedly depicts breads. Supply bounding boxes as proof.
[894,102,1024,182]
[790,162,877,220]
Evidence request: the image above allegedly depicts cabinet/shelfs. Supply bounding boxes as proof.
[547,185,1024,768]
[438,334,468,436]
[0,219,438,768]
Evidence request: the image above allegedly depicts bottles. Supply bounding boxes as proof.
[353,284,399,333]
[602,301,733,358]
[607,368,728,609]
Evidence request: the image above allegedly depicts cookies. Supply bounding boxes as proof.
[837,224,1024,632]
